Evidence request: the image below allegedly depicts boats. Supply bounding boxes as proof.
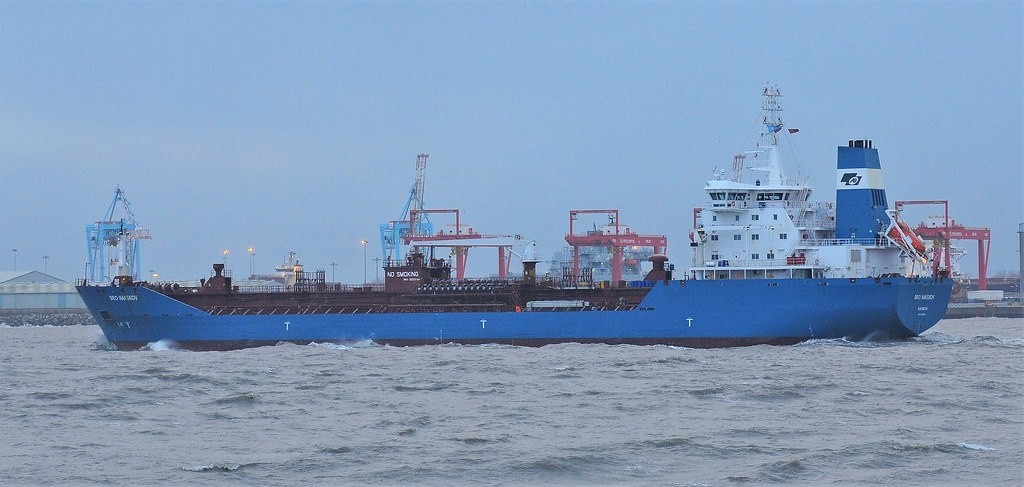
[688,81,932,278]
[71,139,949,351]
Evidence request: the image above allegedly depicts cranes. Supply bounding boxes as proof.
[380,153,433,291]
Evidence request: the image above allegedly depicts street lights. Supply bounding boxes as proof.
[224,251,228,277]
[362,240,369,286]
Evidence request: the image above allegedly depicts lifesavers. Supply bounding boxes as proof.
[804,234,809,239]
[790,214,793,221]
